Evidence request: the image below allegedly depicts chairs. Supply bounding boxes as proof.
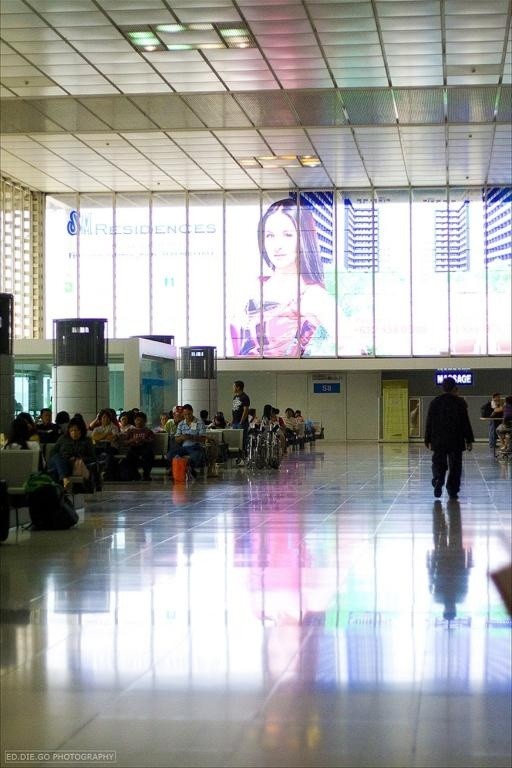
[0,416,325,543]
[496,427,512,464]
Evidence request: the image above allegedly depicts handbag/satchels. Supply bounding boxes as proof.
[24,473,79,531]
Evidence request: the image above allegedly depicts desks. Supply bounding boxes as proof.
[481,416,505,464]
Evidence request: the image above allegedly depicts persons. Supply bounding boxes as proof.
[232,198,356,357]
[0,408,92,489]
[228,380,250,467]
[494,396,512,449]
[89,406,156,483]
[422,378,475,502]
[157,403,226,479]
[261,405,304,452]
[480,392,502,449]
[427,503,473,620]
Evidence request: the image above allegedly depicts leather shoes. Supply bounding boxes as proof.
[434,479,442,497]
